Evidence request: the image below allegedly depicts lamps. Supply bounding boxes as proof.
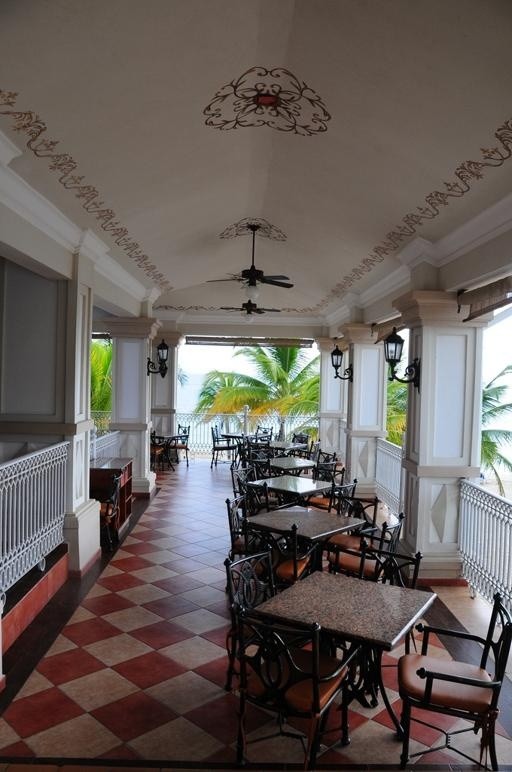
[330,345,354,383]
[242,310,253,321]
[245,273,260,299]
[383,327,420,388]
[147,338,168,378]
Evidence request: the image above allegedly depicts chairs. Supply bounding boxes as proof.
[150,423,189,472]
[395,594,512,770]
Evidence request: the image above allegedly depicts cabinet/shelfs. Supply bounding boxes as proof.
[90,456,133,549]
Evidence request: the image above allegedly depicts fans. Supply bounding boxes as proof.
[221,298,281,315]
[206,224,294,289]
